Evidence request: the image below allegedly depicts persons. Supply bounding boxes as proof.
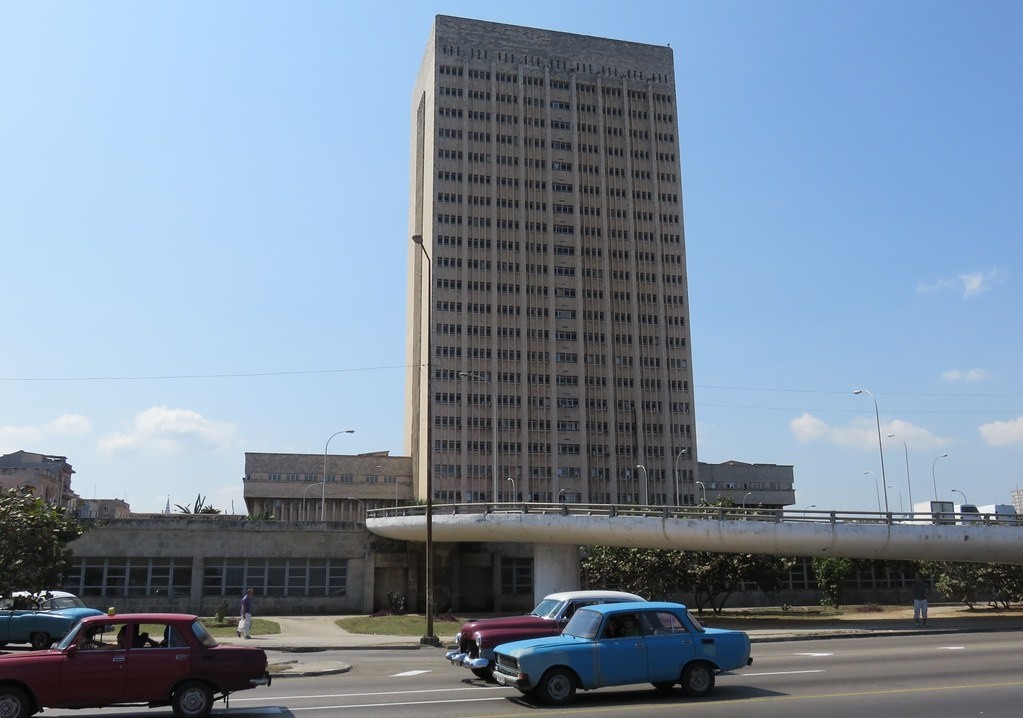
[147,626,168,648]
[911,573,930,628]
[604,617,624,639]
[618,614,640,637]
[82,625,127,650]
[236,588,254,639]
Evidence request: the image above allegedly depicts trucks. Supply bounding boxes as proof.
[899,500,1018,526]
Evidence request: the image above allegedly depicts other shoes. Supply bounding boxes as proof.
[245,636,251,639]
[236,631,241,637]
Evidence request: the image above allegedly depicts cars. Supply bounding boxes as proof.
[1,607,273,718]
[1,591,115,650]
[447,591,708,684]
[490,599,754,705]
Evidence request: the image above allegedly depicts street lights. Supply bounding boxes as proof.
[460,371,499,509]
[302,483,322,522]
[852,390,967,520]
[320,429,354,522]
[412,233,440,643]
[696,480,707,504]
[556,488,565,504]
[507,477,515,503]
[636,464,648,505]
[742,493,751,511]
[348,497,365,523]
[803,504,815,524]
[675,449,687,507]
[376,465,399,510]
